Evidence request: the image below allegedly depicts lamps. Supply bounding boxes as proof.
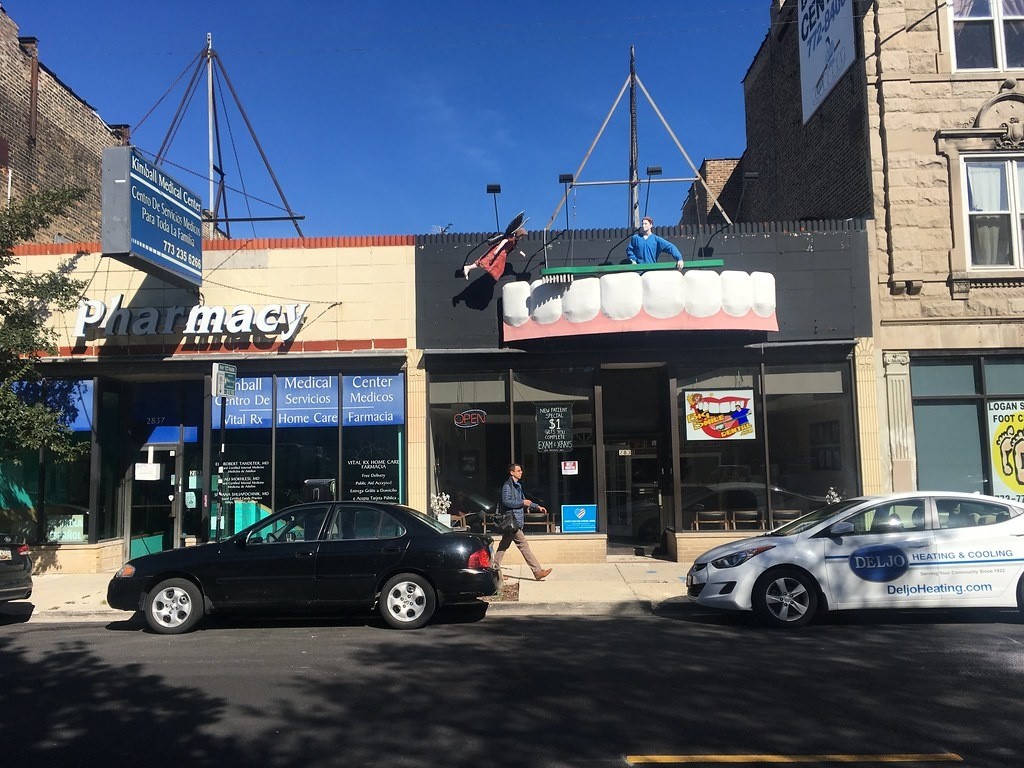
[485,166,760,236]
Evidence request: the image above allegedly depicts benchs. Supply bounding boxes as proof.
[481,512,555,535]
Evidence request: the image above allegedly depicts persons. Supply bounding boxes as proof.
[626,217,683,276]
[464,228,529,281]
[494,464,553,581]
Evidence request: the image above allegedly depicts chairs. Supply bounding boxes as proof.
[731,511,766,529]
[912,507,925,530]
[773,509,803,529]
[885,513,904,531]
[967,513,1009,525]
[692,511,729,529]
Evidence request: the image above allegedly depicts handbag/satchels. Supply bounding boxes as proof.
[492,513,519,535]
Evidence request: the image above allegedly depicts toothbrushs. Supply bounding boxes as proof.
[539,259,725,286]
[715,412,752,430]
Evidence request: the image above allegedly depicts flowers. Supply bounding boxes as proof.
[430,491,451,515]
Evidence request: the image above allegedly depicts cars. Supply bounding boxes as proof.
[607,481,835,545]
[452,487,547,521]
[685,489,1024,629]
[106,500,504,635]
[0,531,34,602]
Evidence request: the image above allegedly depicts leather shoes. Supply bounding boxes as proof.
[535,568,552,581]
[503,575,509,580]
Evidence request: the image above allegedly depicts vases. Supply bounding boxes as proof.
[438,513,450,528]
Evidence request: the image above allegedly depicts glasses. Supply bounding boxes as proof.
[514,469,522,473]
